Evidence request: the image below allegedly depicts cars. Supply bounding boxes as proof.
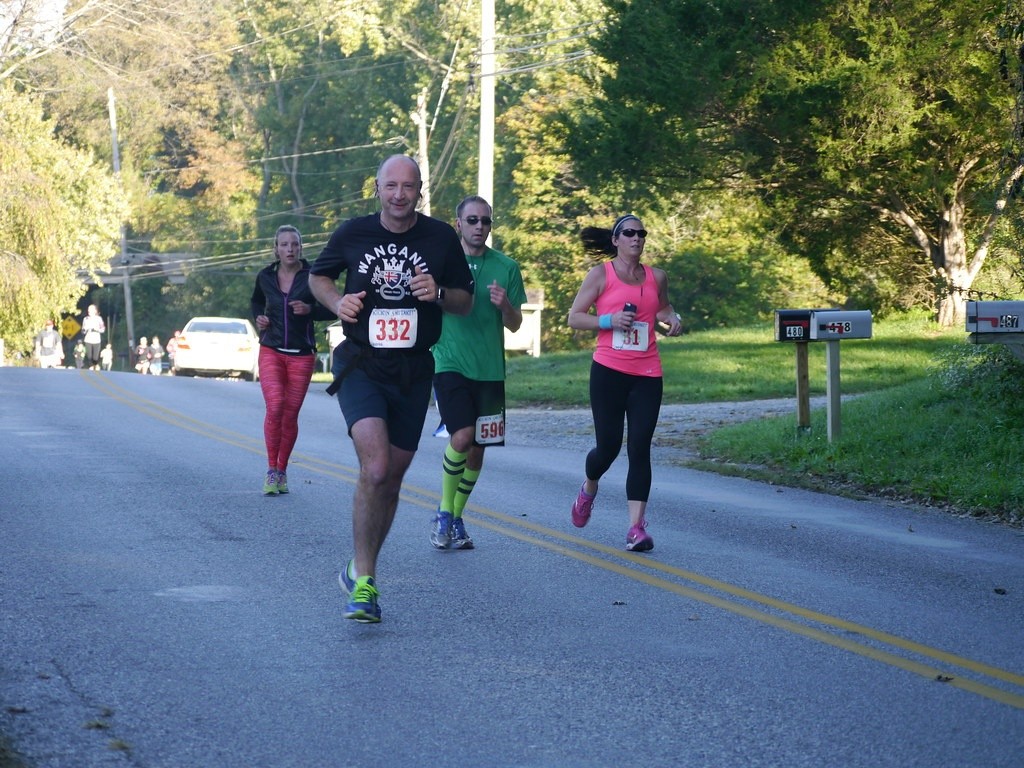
[173,317,260,382]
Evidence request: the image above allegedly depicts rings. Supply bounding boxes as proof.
[677,331,679,334]
[424,288,428,294]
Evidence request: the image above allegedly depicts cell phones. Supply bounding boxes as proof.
[623,301,637,327]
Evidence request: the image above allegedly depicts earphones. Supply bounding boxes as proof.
[419,192,422,197]
[458,225,460,227]
[375,189,378,196]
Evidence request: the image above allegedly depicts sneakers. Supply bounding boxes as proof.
[342,575,379,620]
[263,469,279,495]
[277,472,288,493]
[450,516,473,548]
[338,559,355,594]
[430,505,453,549]
[626,519,654,550]
[571,480,599,527]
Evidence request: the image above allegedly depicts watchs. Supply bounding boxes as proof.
[674,313,682,321]
[437,285,444,299]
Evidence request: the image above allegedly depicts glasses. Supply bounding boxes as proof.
[619,229,647,238]
[461,216,492,225]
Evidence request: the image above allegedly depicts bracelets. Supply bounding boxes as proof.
[599,314,612,329]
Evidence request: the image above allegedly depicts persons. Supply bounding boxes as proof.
[429,196,527,548]
[34,304,181,376]
[308,153,476,624]
[571,215,682,551]
[250,224,338,496]
